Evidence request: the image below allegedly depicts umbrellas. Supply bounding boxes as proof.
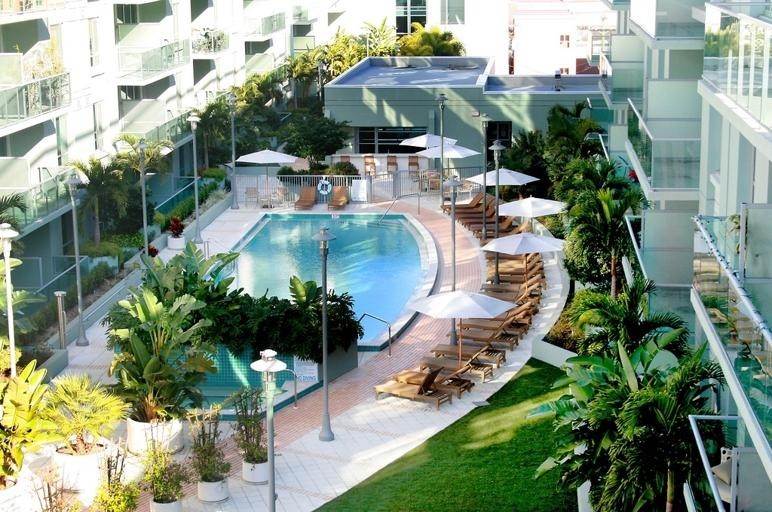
[416,144,488,176]
[235,147,298,196]
[398,134,459,170]
[481,233,569,257]
[493,192,573,220]
[407,287,518,361]
[467,164,543,198]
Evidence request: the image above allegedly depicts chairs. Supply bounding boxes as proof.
[294,186,318,210]
[693,259,772,387]
[328,186,350,210]
[245,186,289,208]
[373,191,546,412]
[709,446,740,504]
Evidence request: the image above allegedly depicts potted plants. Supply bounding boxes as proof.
[228,385,281,485]
[126,440,189,512]
[35,369,133,490]
[103,285,218,455]
[168,217,185,249]
[184,401,230,502]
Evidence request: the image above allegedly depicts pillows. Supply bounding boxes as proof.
[711,458,732,485]
